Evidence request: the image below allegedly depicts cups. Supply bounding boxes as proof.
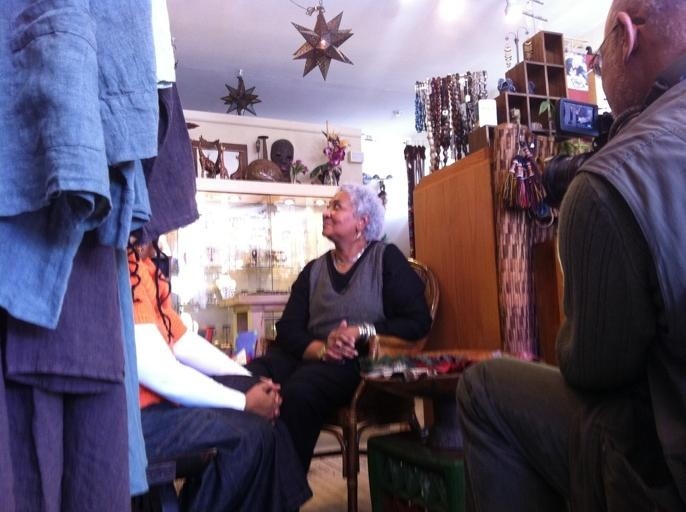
[355,334,379,374]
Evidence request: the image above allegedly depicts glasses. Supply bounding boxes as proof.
[589,18,645,75]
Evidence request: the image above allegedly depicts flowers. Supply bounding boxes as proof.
[308,129,352,179]
[290,160,308,177]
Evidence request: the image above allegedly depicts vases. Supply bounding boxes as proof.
[291,173,297,184]
[324,169,337,185]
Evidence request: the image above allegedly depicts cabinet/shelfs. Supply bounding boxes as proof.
[469,30,569,152]
[413,148,562,355]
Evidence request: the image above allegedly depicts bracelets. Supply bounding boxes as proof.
[356,322,380,342]
[315,344,328,362]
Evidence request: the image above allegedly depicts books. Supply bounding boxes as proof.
[231,330,267,364]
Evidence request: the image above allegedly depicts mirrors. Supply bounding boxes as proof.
[194,140,247,180]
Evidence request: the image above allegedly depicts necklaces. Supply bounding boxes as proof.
[410,70,488,173]
[334,242,369,266]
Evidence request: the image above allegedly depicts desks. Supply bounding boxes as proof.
[351,351,509,512]
[217,294,291,355]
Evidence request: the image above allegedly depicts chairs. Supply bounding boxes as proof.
[146,458,179,512]
[320,256,441,511]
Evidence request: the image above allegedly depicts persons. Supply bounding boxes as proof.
[239,184,435,476]
[271,138,295,183]
[455,1,685,509]
[126,225,284,512]
